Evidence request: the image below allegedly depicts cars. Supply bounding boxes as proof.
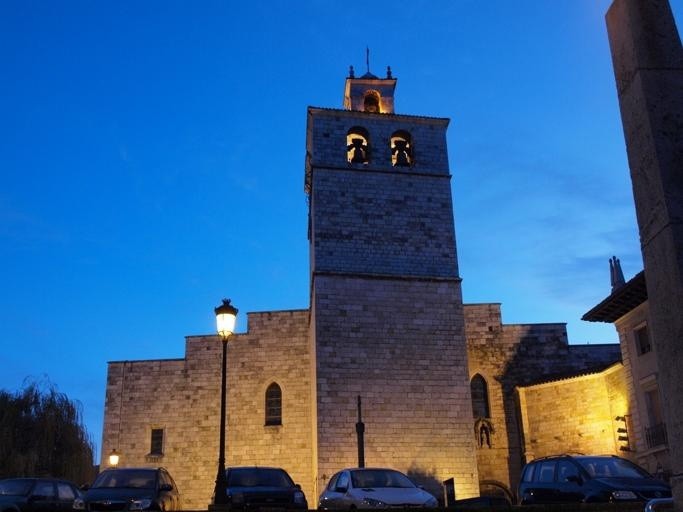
[319,468,439,512]
[0,478,85,512]
[211,466,308,512]
[73,467,182,512]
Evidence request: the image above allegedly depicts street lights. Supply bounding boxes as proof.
[214,297,239,512]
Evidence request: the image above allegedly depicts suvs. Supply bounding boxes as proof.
[517,452,674,512]
